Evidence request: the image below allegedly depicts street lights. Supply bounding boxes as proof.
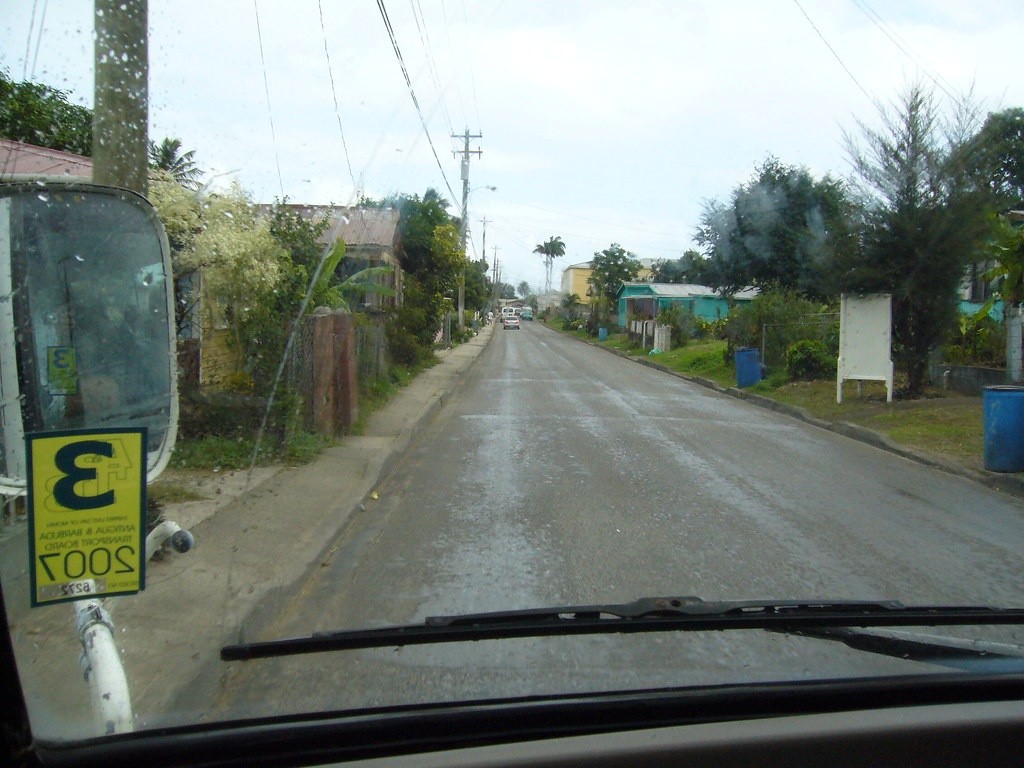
[457,183,497,326]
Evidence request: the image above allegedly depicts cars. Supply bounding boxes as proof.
[514,308,521,316]
[503,316,520,330]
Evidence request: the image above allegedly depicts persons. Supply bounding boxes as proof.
[487,311,493,325]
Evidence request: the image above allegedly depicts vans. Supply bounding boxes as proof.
[501,307,516,322]
[521,306,534,322]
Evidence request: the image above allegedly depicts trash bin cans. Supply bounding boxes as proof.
[734,344,762,389]
[598,326,608,341]
[980,378,1024,475]
